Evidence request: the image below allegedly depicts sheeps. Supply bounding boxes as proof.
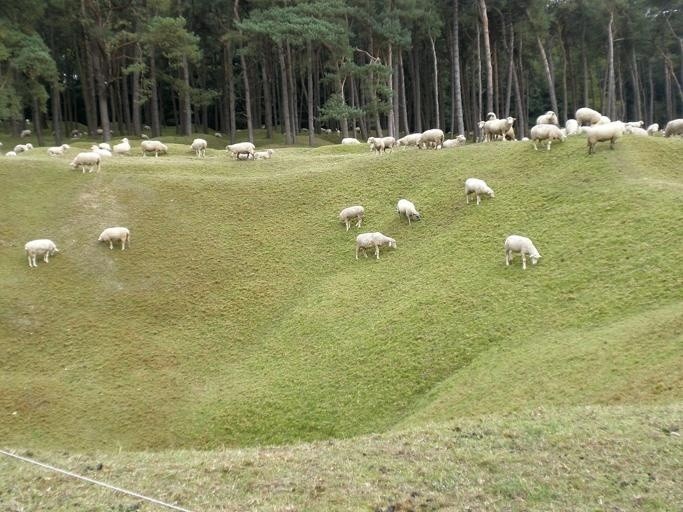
[225,141,273,161]
[563,118,582,136]
[97,225,133,253]
[139,139,168,158]
[536,111,560,124]
[503,233,543,272]
[463,176,496,207]
[45,137,131,175]
[366,136,396,155]
[570,107,618,126]
[354,231,398,262]
[528,124,566,153]
[440,134,467,149]
[6,143,33,157]
[23,236,61,269]
[337,205,365,232]
[190,137,208,159]
[396,127,445,150]
[663,118,683,138]
[396,197,420,226]
[625,119,660,138]
[476,112,520,142]
[580,121,627,155]
